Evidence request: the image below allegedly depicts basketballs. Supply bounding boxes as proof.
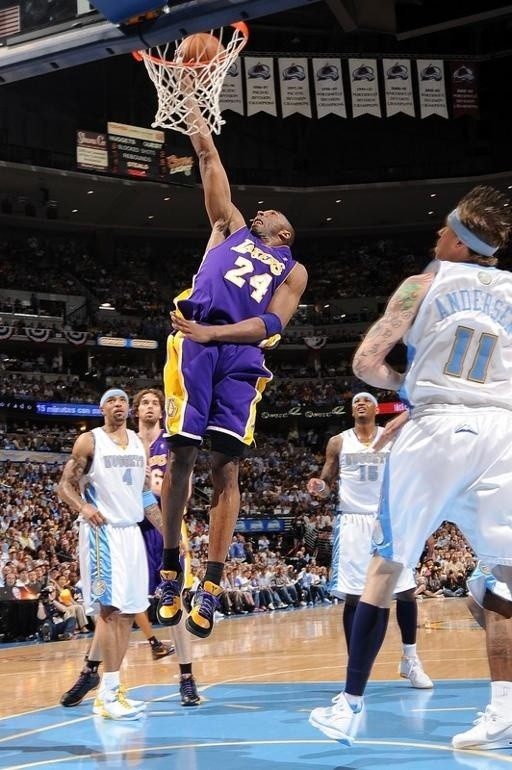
[179,33,231,90]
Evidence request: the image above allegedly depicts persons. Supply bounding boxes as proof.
[1,182,512,752]
[154,57,309,637]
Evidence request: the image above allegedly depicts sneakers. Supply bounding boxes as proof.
[308,691,366,748]
[226,596,341,615]
[56,667,101,707]
[178,672,202,707]
[56,625,90,642]
[155,566,185,628]
[397,655,435,690]
[150,642,175,662]
[92,684,148,723]
[451,705,512,752]
[184,577,224,639]
